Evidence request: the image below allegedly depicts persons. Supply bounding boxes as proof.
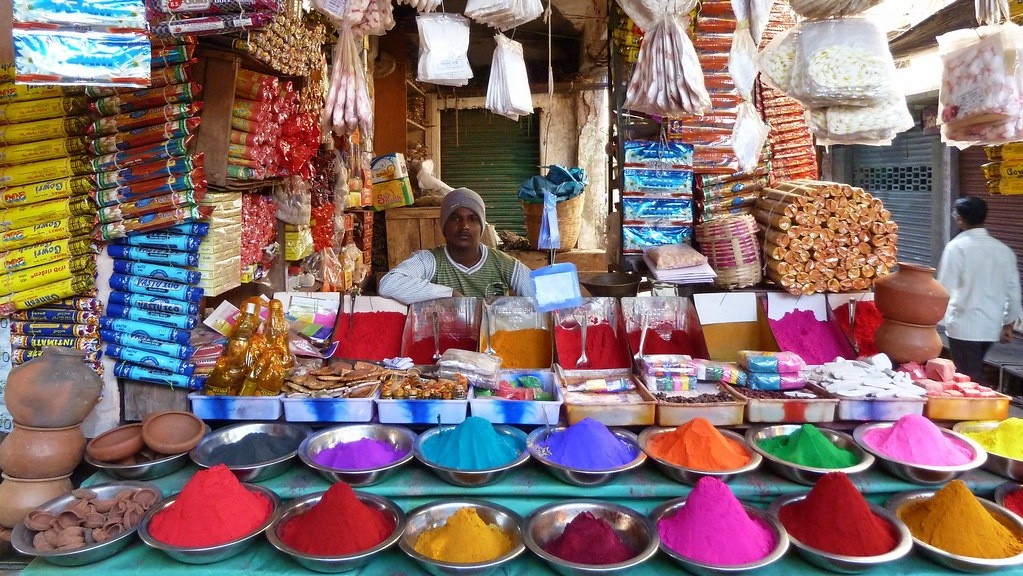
[378,187,536,306]
[936,196,1023,384]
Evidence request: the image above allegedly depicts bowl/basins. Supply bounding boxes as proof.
[578,272,641,299]
[10,421,1023,571]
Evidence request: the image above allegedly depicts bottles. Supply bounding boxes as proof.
[873,262,951,364]
[0,345,104,557]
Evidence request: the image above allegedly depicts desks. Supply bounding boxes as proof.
[386,205,445,273]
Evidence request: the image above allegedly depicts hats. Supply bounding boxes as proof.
[440,187,486,238]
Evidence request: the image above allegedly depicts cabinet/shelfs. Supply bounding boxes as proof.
[374,60,430,169]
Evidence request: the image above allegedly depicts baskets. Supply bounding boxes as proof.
[520,191,585,252]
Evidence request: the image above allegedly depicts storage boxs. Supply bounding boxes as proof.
[370,178,415,211]
[164,288,1013,427]
[623,138,698,267]
[372,154,407,182]
